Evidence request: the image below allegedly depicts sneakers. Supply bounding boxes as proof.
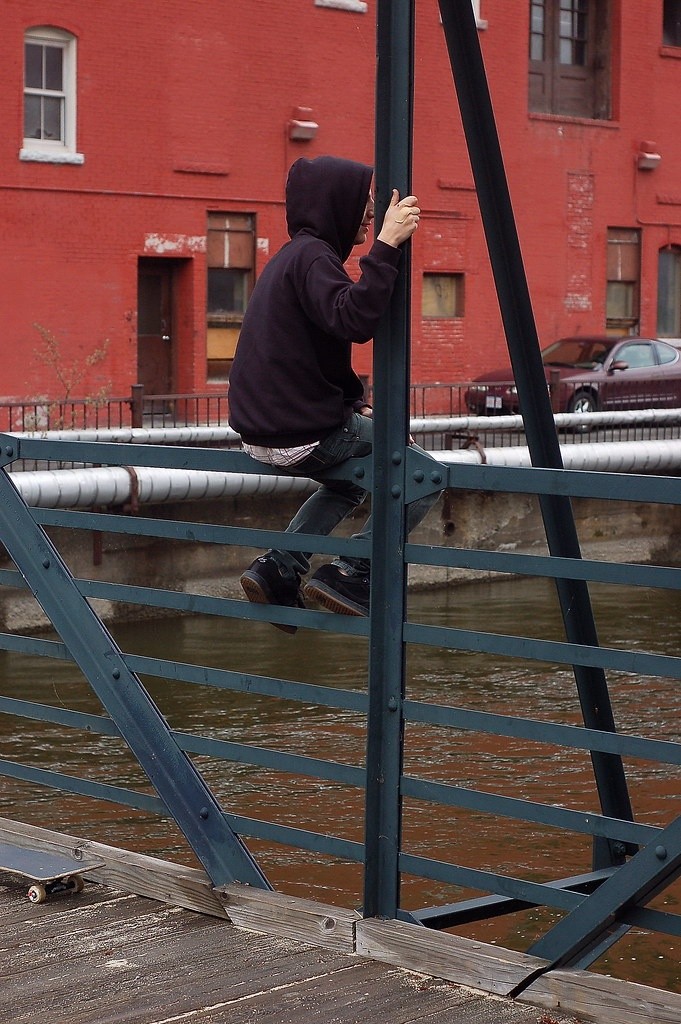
[303,562,371,617]
[240,555,300,634]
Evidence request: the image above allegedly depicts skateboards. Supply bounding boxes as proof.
[0,843,107,904]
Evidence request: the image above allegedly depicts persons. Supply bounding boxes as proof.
[226,155,444,634]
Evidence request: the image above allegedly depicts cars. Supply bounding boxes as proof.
[464,334,681,434]
